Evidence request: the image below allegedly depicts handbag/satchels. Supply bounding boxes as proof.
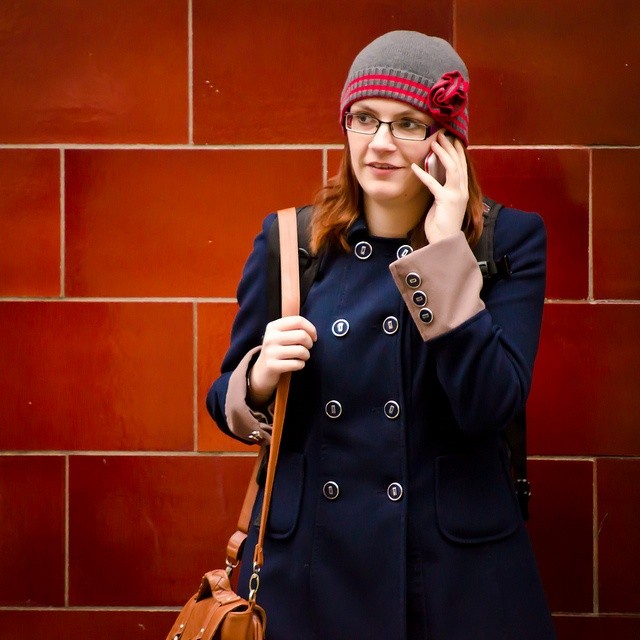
[166,207,300,640]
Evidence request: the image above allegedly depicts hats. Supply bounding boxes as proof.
[340,30,469,148]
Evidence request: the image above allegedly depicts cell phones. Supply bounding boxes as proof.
[425,135,454,184]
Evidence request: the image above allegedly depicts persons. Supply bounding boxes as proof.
[205,29,558,639]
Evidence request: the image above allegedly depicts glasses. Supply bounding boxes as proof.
[344,111,442,141]
